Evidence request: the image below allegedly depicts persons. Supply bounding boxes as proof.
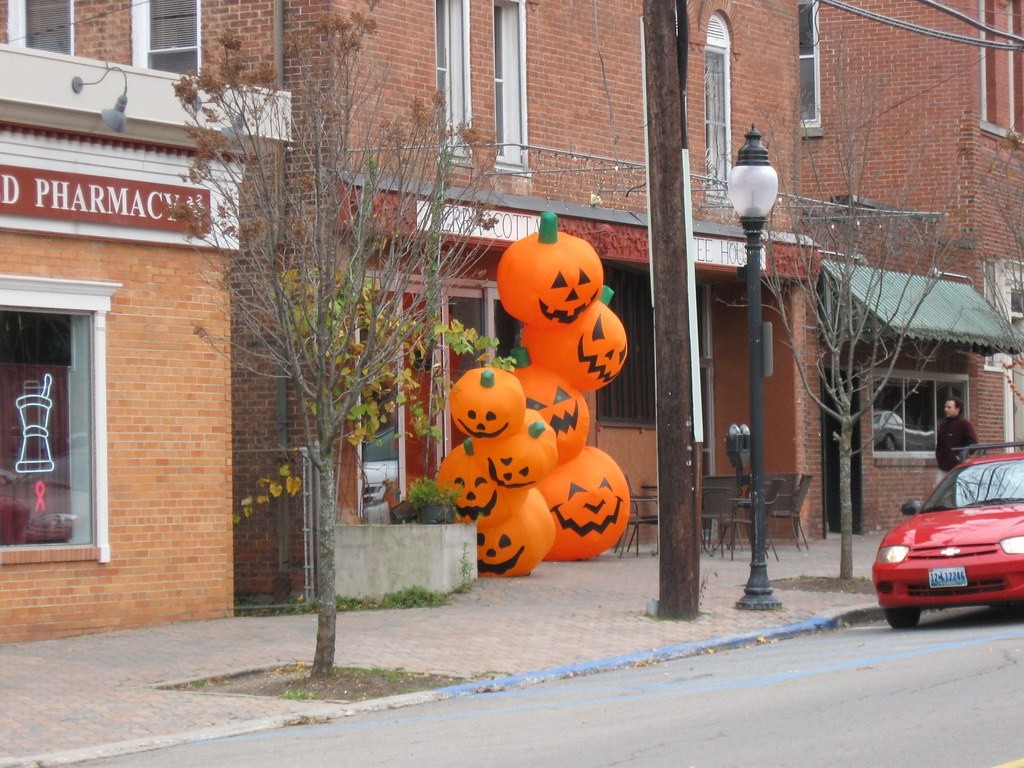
[934,396,980,486]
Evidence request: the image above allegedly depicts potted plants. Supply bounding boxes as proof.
[407,477,463,524]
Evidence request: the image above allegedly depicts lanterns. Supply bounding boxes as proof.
[436,209,632,578]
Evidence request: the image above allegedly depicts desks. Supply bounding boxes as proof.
[640,485,732,558]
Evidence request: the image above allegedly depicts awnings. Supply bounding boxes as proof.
[820,257,1024,354]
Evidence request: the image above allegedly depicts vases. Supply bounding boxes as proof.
[390,499,419,525]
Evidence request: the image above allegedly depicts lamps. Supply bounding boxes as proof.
[72,67,128,133]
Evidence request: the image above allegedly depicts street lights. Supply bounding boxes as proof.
[727,124,782,613]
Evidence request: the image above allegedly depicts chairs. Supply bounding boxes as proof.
[761,472,813,554]
[604,471,786,563]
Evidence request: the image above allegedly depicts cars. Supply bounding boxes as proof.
[873,409,905,453]
[871,439,1024,629]
